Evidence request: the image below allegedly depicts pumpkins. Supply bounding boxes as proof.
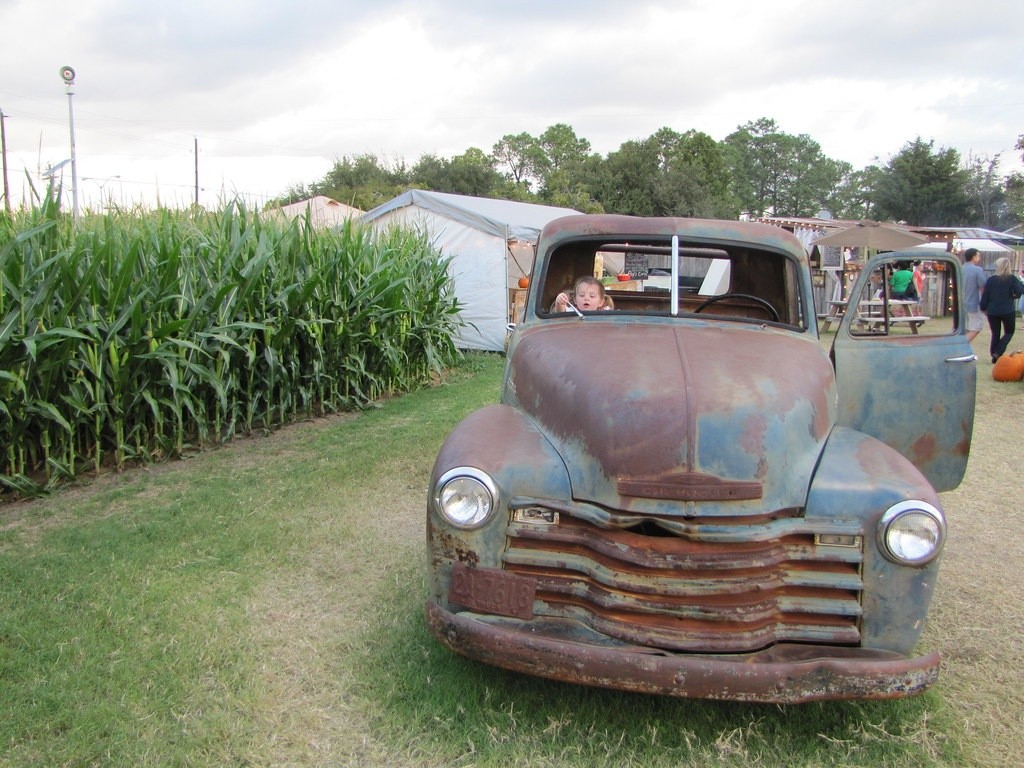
[518,274,530,288]
[992,350,1024,382]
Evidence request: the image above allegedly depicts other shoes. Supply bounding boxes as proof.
[991,358,997,363]
[992,350,1002,359]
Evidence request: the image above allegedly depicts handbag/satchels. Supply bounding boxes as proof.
[905,279,916,297]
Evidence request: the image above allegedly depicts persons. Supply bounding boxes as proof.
[886,256,923,317]
[979,258,1024,365]
[555,277,614,312]
[960,248,987,343]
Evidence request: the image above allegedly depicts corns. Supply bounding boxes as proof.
[38,323,272,378]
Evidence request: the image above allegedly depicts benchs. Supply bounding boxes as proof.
[859,316,930,335]
[817,311,882,334]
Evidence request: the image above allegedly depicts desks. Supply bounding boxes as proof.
[826,300,920,335]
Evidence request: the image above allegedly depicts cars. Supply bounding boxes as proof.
[418,212,977,712]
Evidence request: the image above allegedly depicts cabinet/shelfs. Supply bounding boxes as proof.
[509,287,528,324]
